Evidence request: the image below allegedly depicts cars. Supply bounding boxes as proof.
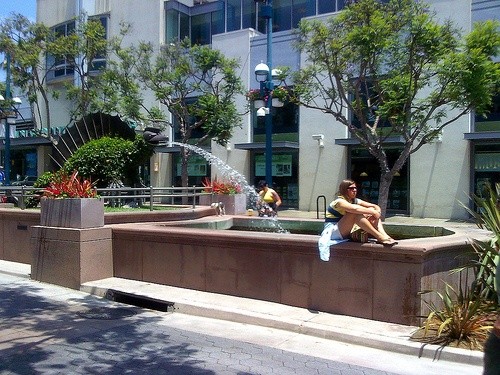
[10,175,37,186]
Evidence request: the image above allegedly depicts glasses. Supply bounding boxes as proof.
[348,187,358,190]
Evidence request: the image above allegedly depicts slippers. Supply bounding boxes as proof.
[376,237,398,245]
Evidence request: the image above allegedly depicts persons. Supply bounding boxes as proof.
[256,181,282,218]
[323,180,398,247]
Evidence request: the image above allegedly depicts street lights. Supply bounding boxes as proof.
[255,0,282,189]
[0,55,22,202]
